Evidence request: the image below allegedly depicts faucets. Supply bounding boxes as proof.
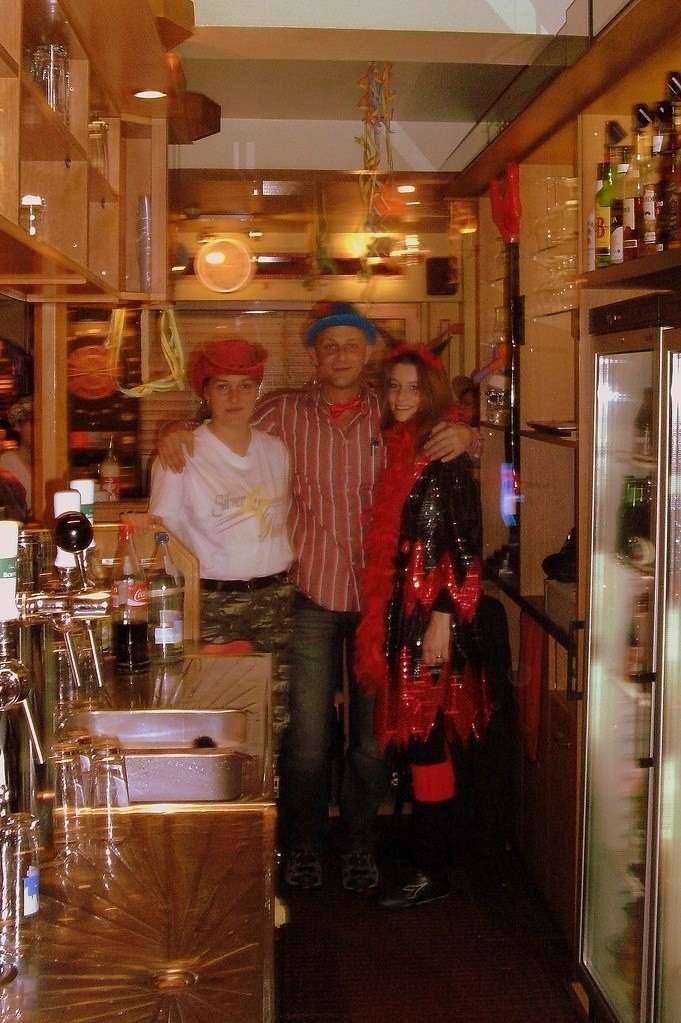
[16,588,111,689]
[0,657,45,766]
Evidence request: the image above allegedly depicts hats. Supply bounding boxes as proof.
[304,304,377,346]
[188,338,268,396]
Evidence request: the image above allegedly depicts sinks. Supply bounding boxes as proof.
[59,709,250,749]
[0,801,277,1023]
[50,753,251,801]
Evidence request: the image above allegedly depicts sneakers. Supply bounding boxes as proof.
[287,847,321,887]
[343,851,379,889]
[371,869,450,906]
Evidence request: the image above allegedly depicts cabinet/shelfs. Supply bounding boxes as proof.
[0,0,221,303]
[0,652,287,1023]
[458,31,679,943]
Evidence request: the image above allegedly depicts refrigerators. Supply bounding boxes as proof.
[579,327,681,1023]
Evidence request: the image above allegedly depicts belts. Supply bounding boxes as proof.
[199,570,286,592]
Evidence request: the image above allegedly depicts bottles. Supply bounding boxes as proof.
[592,71,680,267]
[147,531,185,665]
[615,387,657,678]
[3,811,42,924]
[111,525,149,675]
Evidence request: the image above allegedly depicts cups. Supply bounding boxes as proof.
[46,733,131,841]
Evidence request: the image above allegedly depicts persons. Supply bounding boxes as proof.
[146,339,298,927]
[156,301,485,893]
[451,375,480,428]
[352,342,503,909]
